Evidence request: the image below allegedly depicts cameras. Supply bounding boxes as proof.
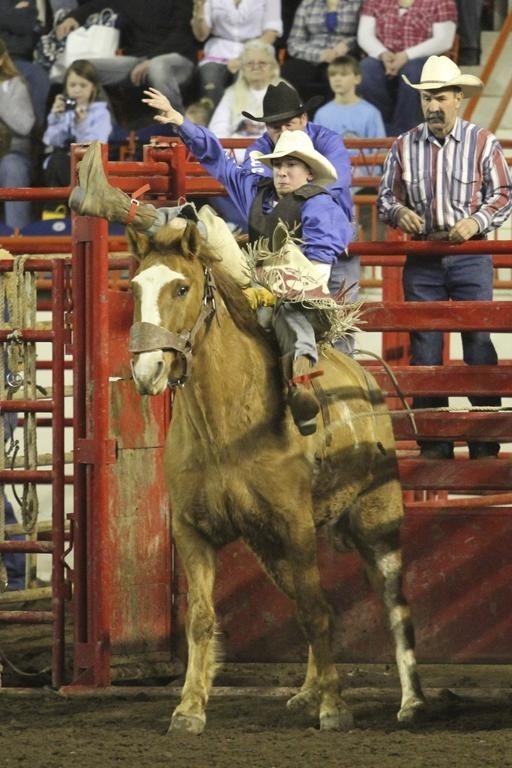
[61,95,81,115]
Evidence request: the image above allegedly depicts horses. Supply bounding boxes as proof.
[125,218,429,737]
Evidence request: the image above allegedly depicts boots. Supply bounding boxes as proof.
[67,138,159,233]
[281,354,322,437]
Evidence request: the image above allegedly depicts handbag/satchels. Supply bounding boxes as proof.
[42,204,68,219]
[62,24,120,69]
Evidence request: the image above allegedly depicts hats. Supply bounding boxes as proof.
[401,53,484,99]
[254,129,338,186]
[242,81,323,124]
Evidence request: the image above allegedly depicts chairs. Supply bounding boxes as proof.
[197,47,285,64]
[447,33,459,65]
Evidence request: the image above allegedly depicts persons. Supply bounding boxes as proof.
[240,81,361,357]
[1,1,483,241]
[376,55,511,459]
[65,87,352,438]
